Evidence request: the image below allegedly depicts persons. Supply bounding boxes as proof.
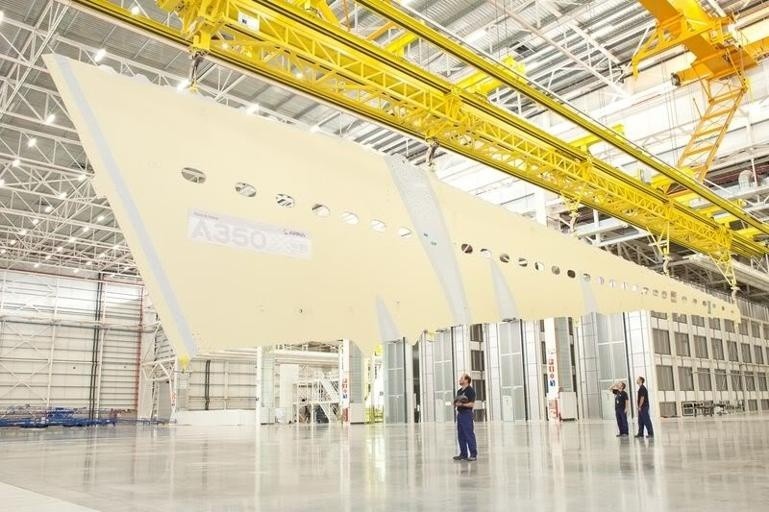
[451,374,479,462]
[619,438,636,482]
[608,381,630,438]
[459,460,479,512]
[638,438,657,499]
[633,376,654,438]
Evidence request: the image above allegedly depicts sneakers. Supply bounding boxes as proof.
[453,456,477,462]
[615,434,654,438]
